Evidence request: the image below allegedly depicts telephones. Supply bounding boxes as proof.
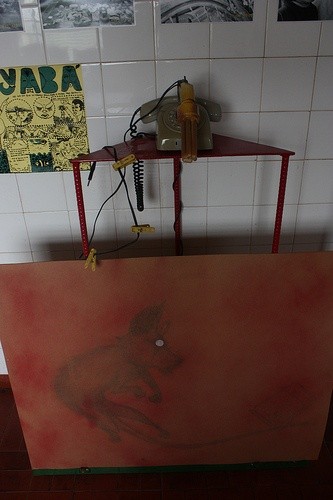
[140,78,221,163]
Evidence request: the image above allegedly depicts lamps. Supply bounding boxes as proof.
[177,83,197,163]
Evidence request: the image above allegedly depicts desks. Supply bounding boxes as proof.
[70,133,298,257]
